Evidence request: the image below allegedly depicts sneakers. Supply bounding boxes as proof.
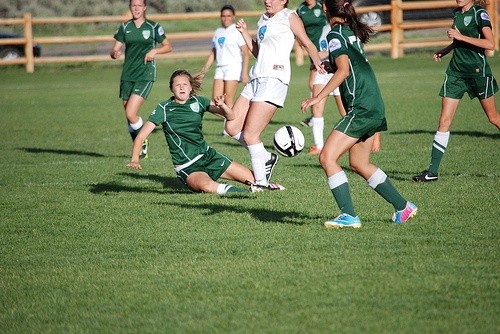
[306,146,321,156]
[391,201,417,223]
[269,182,285,190]
[300,114,313,128]
[249,153,278,192]
[139,139,148,160]
[412,172,438,182]
[324,213,361,228]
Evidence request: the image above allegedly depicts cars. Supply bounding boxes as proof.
[350,0,460,38]
[0,32,40,61]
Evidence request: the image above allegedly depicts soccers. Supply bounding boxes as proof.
[273,124,306,158]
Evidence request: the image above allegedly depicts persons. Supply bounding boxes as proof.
[413,0,500,181]
[125,70,286,199]
[294,0,339,127]
[110,0,173,161]
[304,0,380,156]
[193,4,250,137]
[224,0,326,193]
[300,0,418,229]
